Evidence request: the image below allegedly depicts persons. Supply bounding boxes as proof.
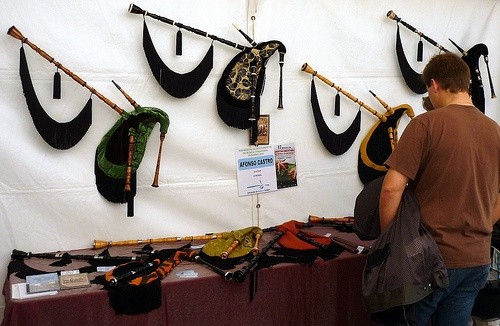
[378,51,500,326]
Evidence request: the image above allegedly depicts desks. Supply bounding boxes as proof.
[0,226,379,326]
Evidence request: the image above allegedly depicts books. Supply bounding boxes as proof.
[24,272,90,295]
